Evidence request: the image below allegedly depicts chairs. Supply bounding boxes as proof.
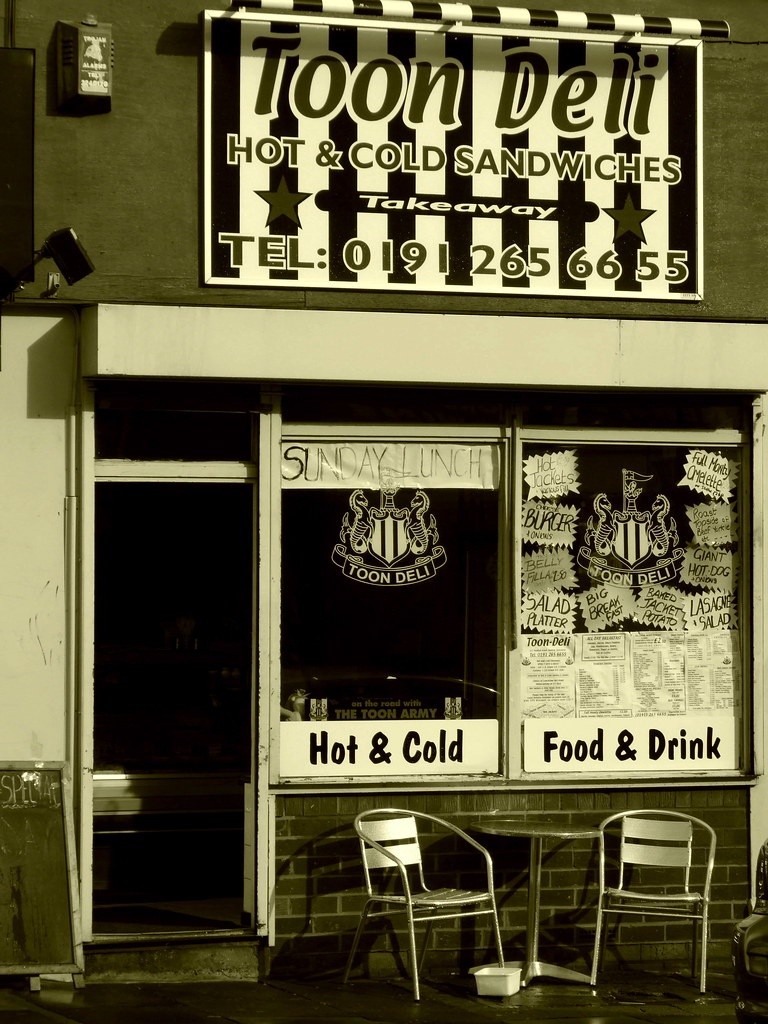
[589,809,718,995]
[343,806,504,1002]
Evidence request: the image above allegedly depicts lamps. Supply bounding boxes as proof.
[35,227,95,285]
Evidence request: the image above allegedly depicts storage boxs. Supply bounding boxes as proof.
[473,966,523,997]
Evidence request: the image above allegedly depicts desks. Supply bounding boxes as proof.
[469,820,603,995]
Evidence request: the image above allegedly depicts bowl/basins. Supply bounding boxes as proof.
[474,966,522,995]
